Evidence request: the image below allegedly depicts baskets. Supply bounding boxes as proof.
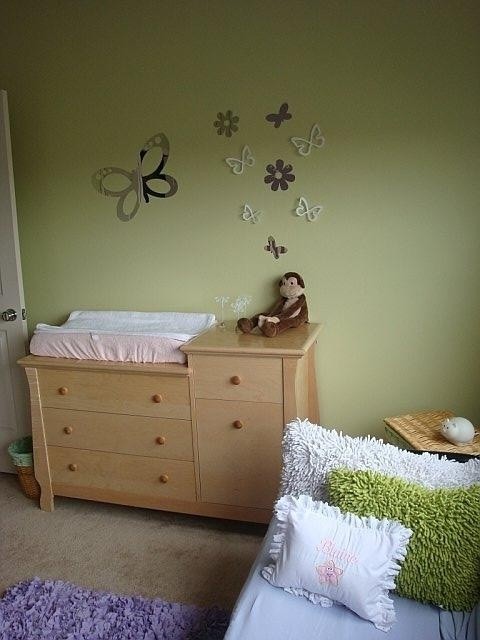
[6,432,41,500]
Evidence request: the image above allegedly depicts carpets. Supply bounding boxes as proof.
[0,576,228,639]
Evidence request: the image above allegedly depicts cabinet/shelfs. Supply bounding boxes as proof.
[16,320,324,524]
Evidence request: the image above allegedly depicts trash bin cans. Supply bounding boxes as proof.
[8,435,41,500]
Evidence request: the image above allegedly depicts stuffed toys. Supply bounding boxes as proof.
[236,270,311,338]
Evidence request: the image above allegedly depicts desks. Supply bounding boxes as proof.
[384,412,480,456]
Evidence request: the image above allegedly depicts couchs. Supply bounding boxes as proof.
[223,451,480,639]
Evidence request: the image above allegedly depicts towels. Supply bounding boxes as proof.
[34,310,215,342]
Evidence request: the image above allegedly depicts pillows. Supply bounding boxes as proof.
[261,494,414,632]
[276,419,480,504]
[326,465,478,613]
